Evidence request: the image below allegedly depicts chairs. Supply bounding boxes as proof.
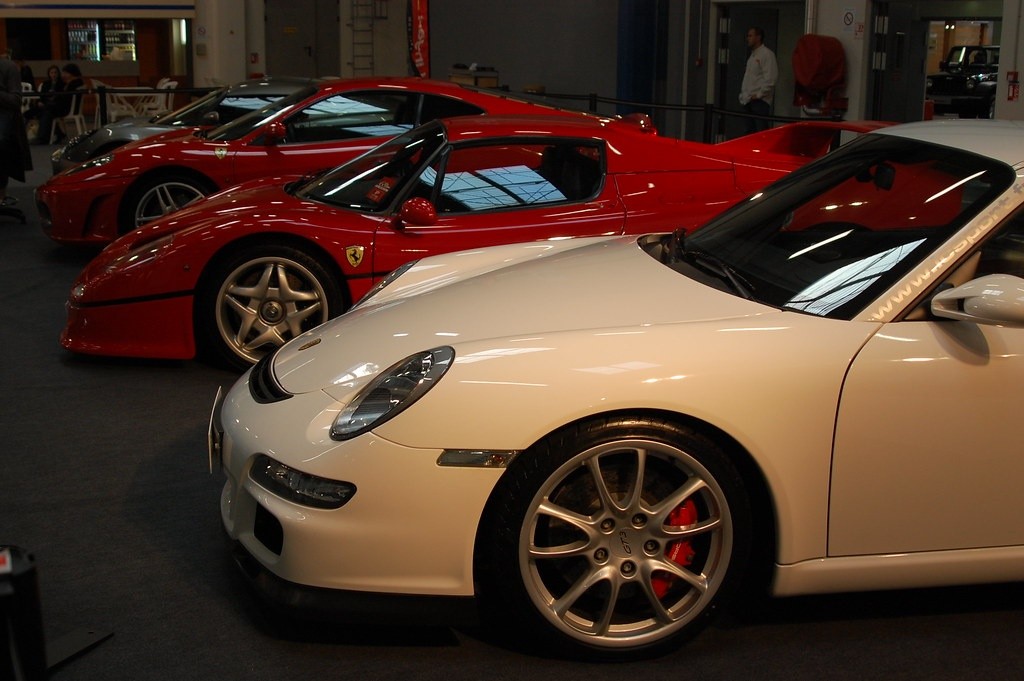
[969,52,989,65]
[20,76,179,144]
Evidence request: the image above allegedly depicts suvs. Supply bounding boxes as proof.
[925,44,999,120]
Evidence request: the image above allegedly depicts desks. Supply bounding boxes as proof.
[112,87,154,120]
[20,89,51,115]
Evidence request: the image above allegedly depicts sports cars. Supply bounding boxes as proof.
[61,114,968,380]
[49,77,309,178]
[207,115,1023,659]
[36,76,658,245]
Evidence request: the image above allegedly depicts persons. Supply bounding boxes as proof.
[12,54,84,146]
[0,57,34,205]
[738,24,779,135]
